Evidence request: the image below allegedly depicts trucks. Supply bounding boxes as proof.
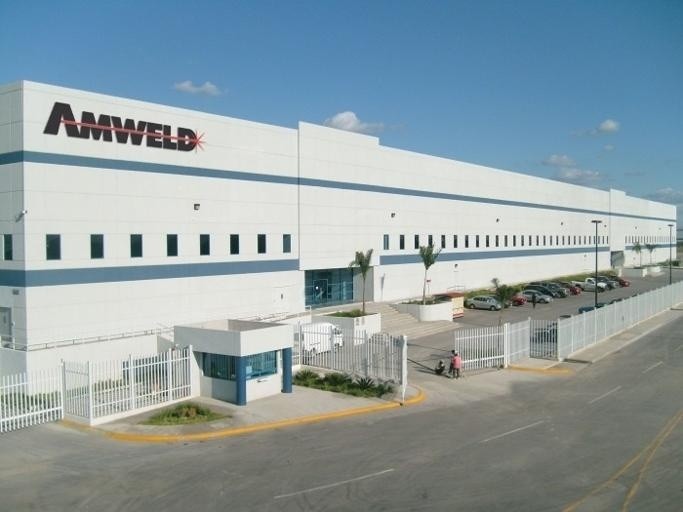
[301,322,347,357]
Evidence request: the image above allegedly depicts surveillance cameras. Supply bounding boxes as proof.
[21,210,25,215]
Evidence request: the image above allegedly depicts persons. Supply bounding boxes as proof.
[447,349,455,373]
[434,359,446,375]
[452,352,461,379]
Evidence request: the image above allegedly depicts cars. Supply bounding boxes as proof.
[465,272,640,344]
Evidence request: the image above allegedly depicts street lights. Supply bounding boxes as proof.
[591,218,602,305]
[666,223,675,286]
